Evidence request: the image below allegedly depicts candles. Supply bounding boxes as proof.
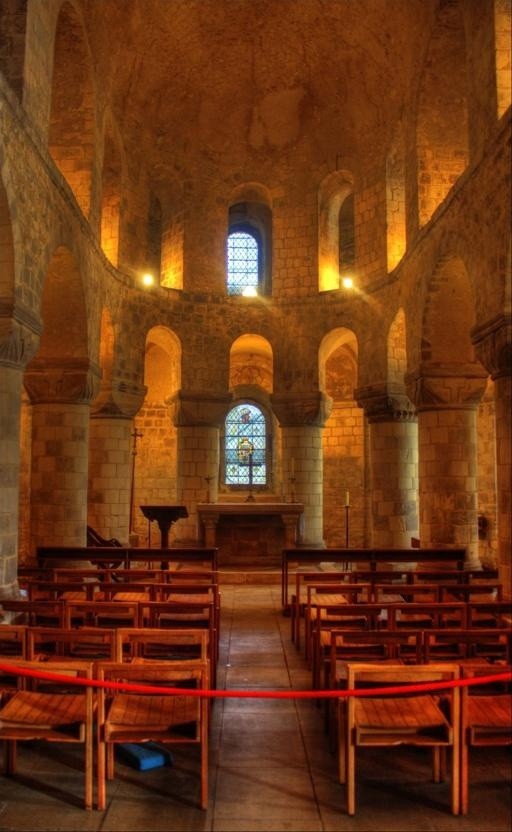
[291,455,295,478]
[344,490,350,505]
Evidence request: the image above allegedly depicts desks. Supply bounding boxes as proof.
[195,503,305,569]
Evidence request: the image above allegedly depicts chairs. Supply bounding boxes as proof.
[290,567,512,817]
[0,565,222,817]
